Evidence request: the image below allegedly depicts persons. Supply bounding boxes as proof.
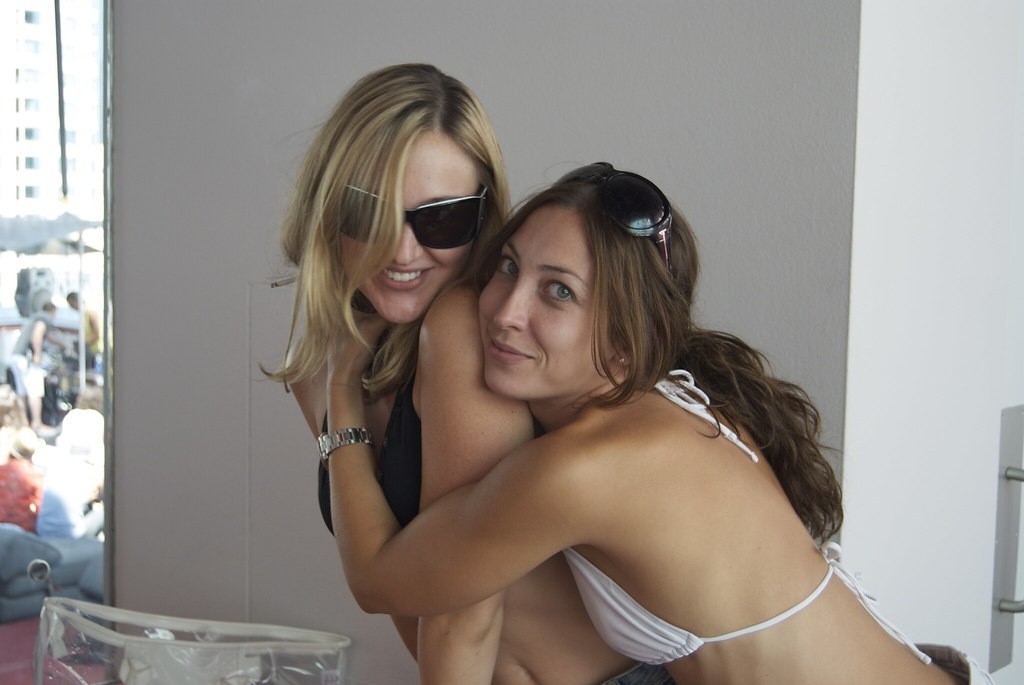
[0,281,108,543]
[252,64,678,685]
[317,162,960,685]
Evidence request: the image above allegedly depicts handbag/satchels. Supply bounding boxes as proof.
[35,586,350,685]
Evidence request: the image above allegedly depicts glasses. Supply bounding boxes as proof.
[315,172,490,249]
[552,162,676,271]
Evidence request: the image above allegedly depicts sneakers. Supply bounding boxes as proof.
[31,422,56,438]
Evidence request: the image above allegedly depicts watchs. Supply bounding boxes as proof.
[317,427,372,472]
